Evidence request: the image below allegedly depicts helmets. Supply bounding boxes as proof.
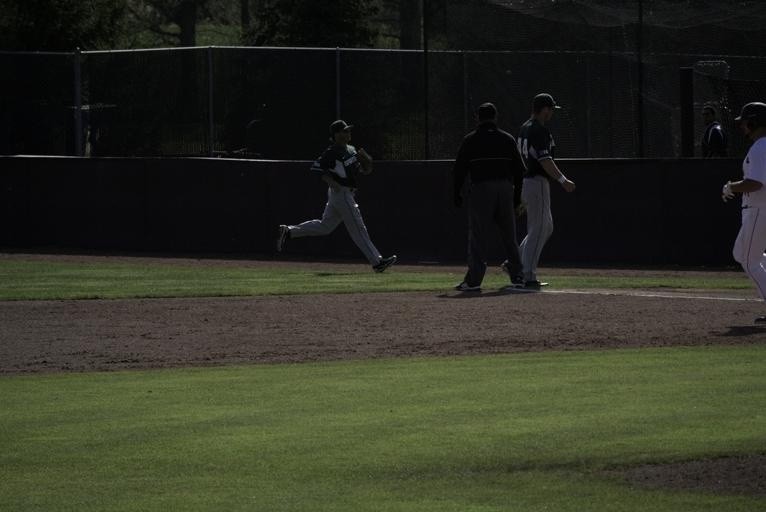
[734,101,766,121]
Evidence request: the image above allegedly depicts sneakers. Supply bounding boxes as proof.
[372,254,398,274]
[501,261,548,287]
[754,315,766,325]
[454,281,482,291]
[276,224,288,253]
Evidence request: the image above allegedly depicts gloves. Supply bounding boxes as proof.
[721,180,735,203]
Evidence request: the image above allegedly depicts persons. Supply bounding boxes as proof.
[450,93,577,291]
[701,100,726,157]
[276,119,397,273]
[721,102,766,324]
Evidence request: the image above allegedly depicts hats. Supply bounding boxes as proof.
[531,93,562,113]
[328,119,354,135]
